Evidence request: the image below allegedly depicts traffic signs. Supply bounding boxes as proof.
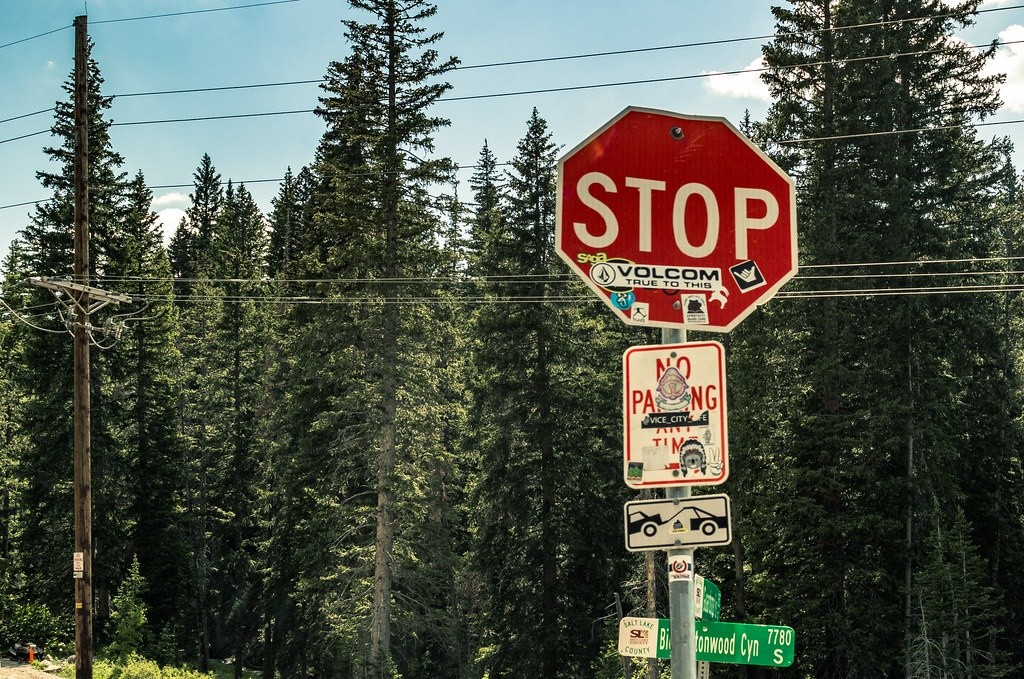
[692,573,722,622]
[618,614,796,670]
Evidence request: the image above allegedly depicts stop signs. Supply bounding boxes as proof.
[555,104,800,337]
